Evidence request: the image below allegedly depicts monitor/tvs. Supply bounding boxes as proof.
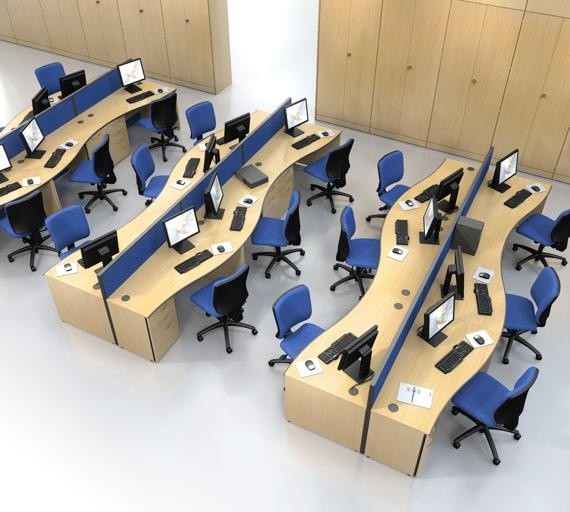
[203,171,225,220]
[284,98,309,138]
[81,228,120,275]
[118,58,145,94]
[417,291,456,347]
[162,206,201,254]
[0,144,12,184]
[203,132,220,176]
[488,149,519,193]
[223,112,250,150]
[20,117,46,159]
[441,245,464,300]
[32,86,51,116]
[59,69,87,97]
[415,167,464,244]
[337,324,380,383]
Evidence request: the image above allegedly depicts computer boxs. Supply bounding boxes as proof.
[451,215,484,256]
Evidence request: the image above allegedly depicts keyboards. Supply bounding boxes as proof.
[183,158,200,178]
[216,137,225,145]
[58,95,64,100]
[0,182,22,198]
[318,332,358,364]
[292,134,320,149]
[474,283,492,315]
[435,341,474,374]
[174,249,213,274]
[19,110,34,126]
[230,206,247,231]
[395,220,408,245]
[126,91,155,104]
[44,148,66,168]
[77,258,84,268]
[504,189,532,208]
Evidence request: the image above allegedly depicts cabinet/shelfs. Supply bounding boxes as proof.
[550,127,570,188]
[368,0,454,148]
[483,10,570,180]
[1,1,232,96]
[460,0,525,12]
[315,1,383,135]
[424,0,524,166]
[526,1,569,19]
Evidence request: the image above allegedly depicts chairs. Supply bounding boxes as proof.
[500,265,561,364]
[190,263,258,354]
[512,207,569,272]
[136,92,187,162]
[364,148,410,223]
[184,100,217,147]
[0,189,58,271]
[34,63,71,93]
[250,189,305,278]
[305,139,355,213]
[45,203,95,260]
[447,366,540,465]
[329,205,380,301]
[129,145,170,206]
[69,133,128,214]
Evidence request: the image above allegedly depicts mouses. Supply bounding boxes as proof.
[27,179,33,185]
[406,200,414,207]
[243,198,253,204]
[217,245,225,253]
[157,89,163,93]
[176,180,185,185]
[65,142,73,147]
[305,360,315,370]
[531,186,540,192]
[393,248,402,254]
[479,272,490,279]
[64,263,72,271]
[322,131,328,136]
[49,98,54,102]
[11,128,15,130]
[205,142,209,147]
[473,335,485,345]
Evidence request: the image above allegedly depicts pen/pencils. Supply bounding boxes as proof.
[411,386,416,403]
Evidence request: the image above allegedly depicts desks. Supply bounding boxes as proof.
[362,167,553,481]
[0,65,182,216]
[43,107,345,359]
[281,154,479,453]
